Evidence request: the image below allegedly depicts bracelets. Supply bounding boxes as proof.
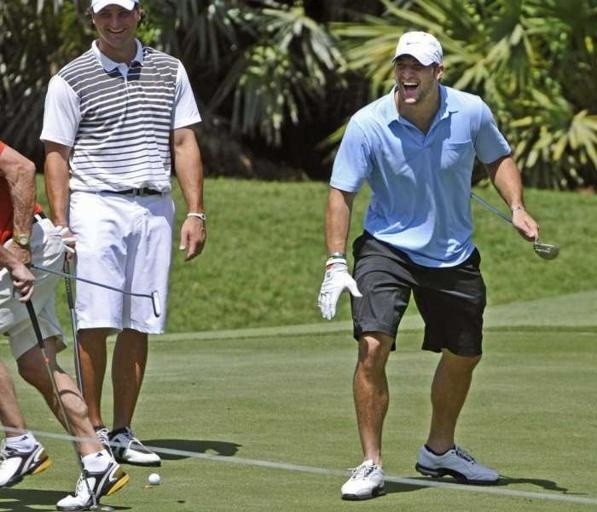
[329,253,346,260]
[188,213,207,221]
[512,206,524,211]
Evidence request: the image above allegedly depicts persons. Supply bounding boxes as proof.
[0,141,129,511]
[318,30,539,499]
[0,246,36,301]
[39,1,206,466]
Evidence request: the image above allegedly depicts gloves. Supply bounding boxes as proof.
[317,263,363,321]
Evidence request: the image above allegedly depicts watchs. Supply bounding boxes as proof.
[11,233,30,247]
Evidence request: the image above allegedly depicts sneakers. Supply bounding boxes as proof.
[0,427,161,511]
[340,459,386,501]
[415,444,499,486]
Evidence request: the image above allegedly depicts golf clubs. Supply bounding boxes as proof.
[24,292,112,511]
[470,191,559,260]
[30,265,160,316]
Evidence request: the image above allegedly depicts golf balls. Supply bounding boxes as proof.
[149,473,161,484]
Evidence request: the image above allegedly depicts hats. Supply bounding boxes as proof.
[88,0,139,14]
[391,30,443,66]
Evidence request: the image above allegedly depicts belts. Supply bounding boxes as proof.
[31,212,47,224]
[101,187,161,197]
